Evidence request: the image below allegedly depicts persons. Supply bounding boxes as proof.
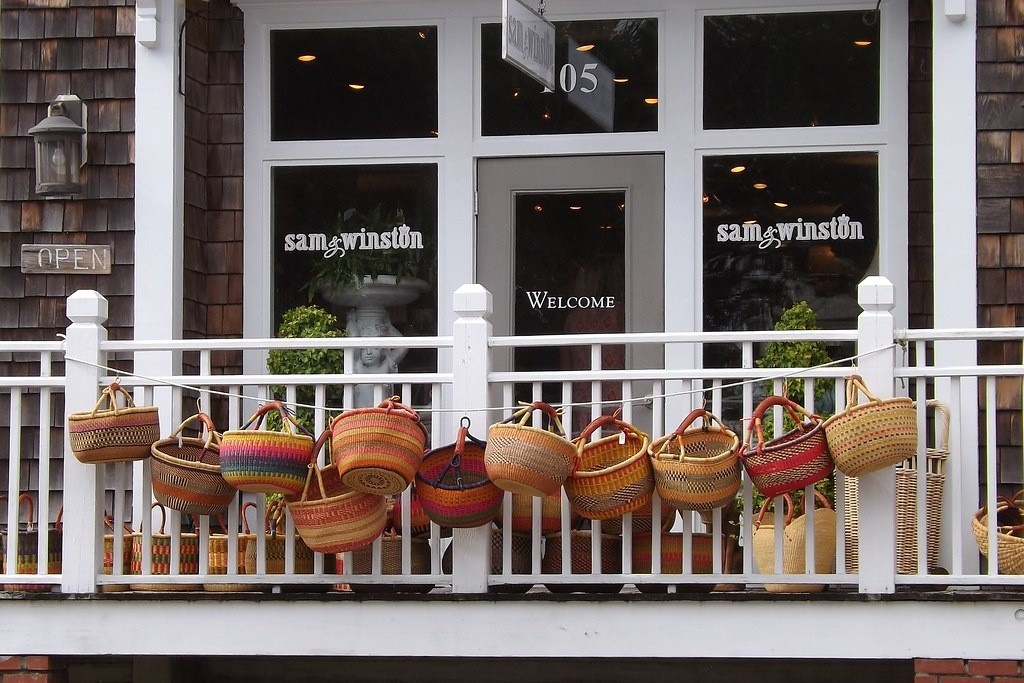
[342,308,408,409]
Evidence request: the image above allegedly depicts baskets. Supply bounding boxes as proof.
[972,491,1024,575]
[149,413,239,515]
[822,378,917,478]
[632,513,726,594]
[541,523,622,593]
[131,503,203,591]
[1,493,68,591]
[67,383,160,465]
[284,430,387,554]
[832,399,950,574]
[562,416,655,520]
[484,401,577,498]
[415,416,504,528]
[203,502,261,593]
[748,490,836,594]
[329,396,428,495]
[246,502,314,575]
[647,409,742,511]
[442,521,532,594]
[494,484,585,536]
[103,509,132,592]
[392,496,433,536]
[349,532,437,593]
[220,400,313,495]
[740,396,835,498]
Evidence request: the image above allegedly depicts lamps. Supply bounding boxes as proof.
[28,100,87,197]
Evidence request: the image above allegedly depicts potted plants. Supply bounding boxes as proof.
[298,205,437,309]
